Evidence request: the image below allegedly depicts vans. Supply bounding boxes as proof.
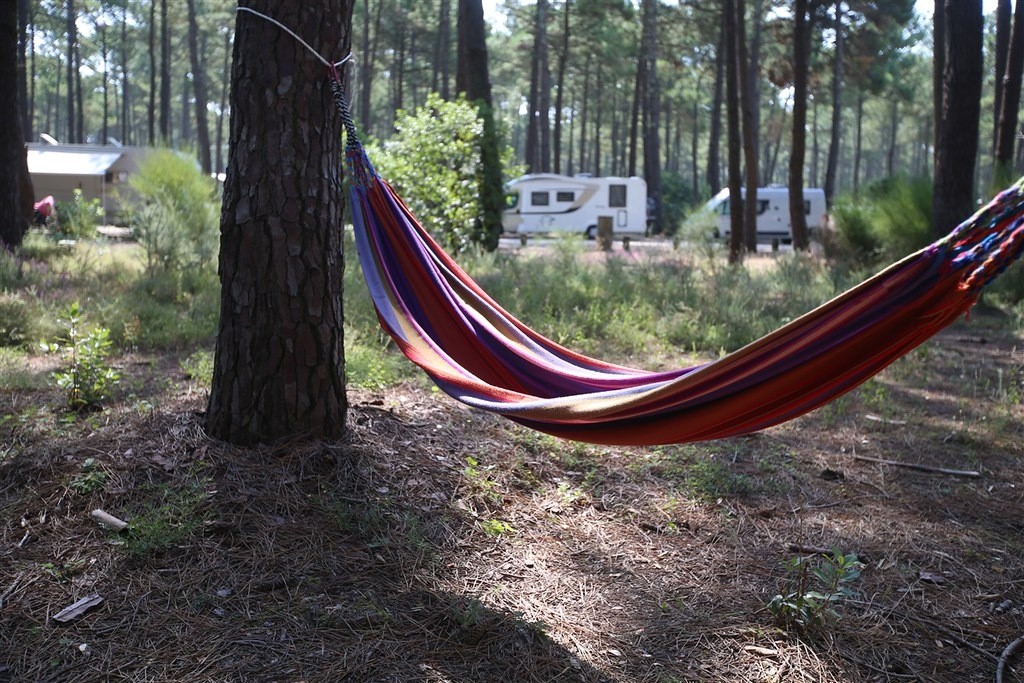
[677,183,829,245]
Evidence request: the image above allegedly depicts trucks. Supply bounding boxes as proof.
[462,173,649,241]
